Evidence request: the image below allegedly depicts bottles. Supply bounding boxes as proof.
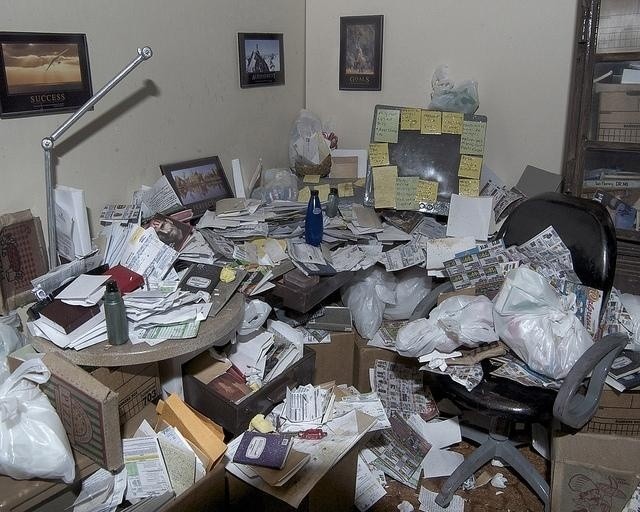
[326,188,339,218]
[304,190,323,247]
[103,281,128,346]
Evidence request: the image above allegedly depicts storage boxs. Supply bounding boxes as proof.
[593,81,640,143]
[548,388,639,510]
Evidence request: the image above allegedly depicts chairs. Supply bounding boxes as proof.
[405,188,628,511]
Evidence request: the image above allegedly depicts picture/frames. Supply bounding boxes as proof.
[236,31,288,89]
[158,155,236,215]
[338,15,384,93]
[0,31,95,121]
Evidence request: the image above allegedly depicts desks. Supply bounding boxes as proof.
[20,260,245,403]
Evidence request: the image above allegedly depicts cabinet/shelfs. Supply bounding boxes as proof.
[555,0,640,300]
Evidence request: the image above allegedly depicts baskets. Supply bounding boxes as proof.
[297,155,331,177]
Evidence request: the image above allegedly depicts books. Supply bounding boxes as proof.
[305,307,353,333]
[347,205,385,236]
[39,298,101,337]
[145,213,202,255]
[584,167,640,233]
[238,266,277,299]
[608,352,640,381]
[232,430,311,486]
[605,372,640,394]
[177,263,224,297]
[292,260,338,279]
[101,264,145,299]
